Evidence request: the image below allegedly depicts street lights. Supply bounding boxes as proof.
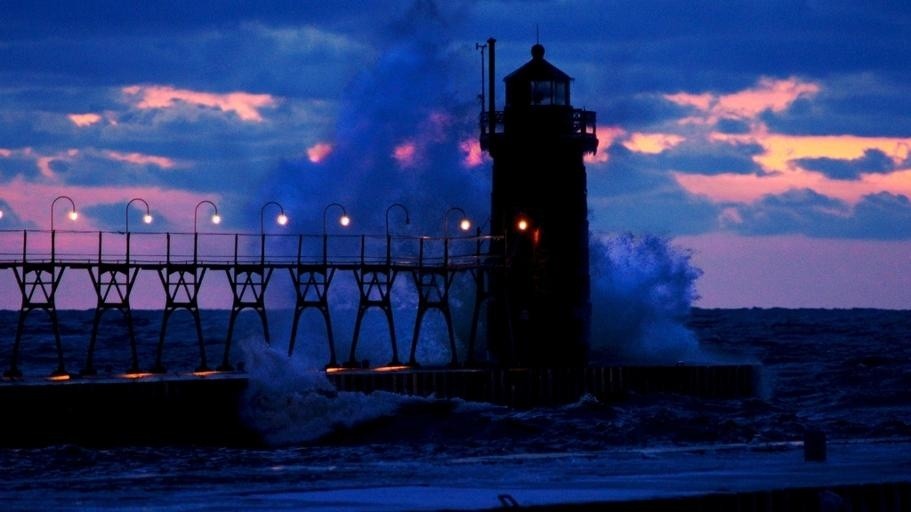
[51,196,78,262]
[323,203,350,264]
[444,207,471,267]
[194,200,221,264]
[126,198,153,264]
[386,203,410,264]
[261,202,288,264]
